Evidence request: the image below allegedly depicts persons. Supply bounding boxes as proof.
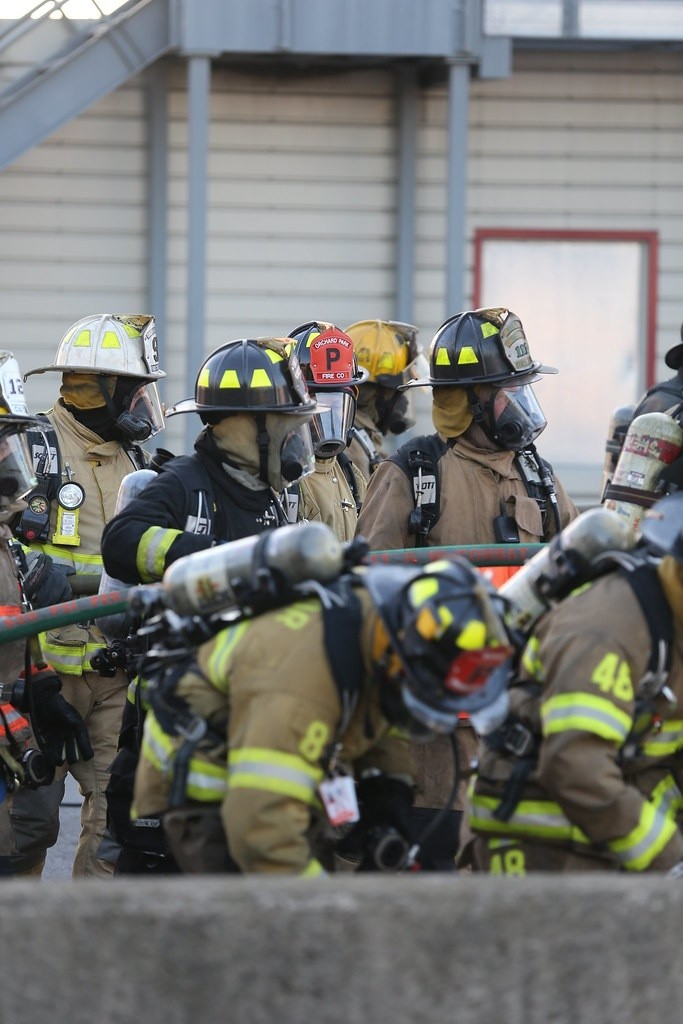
[456,495,683,880]
[120,558,518,890]
[606,324,683,531]
[0,318,431,882]
[349,304,578,558]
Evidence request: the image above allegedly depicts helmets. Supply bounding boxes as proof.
[396,306,560,388]
[287,320,370,388]
[0,349,56,433]
[344,319,430,392]
[366,558,514,736]
[640,491,683,563]
[165,337,332,418]
[23,313,167,383]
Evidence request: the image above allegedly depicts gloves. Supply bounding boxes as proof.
[23,554,76,610]
[32,670,94,767]
